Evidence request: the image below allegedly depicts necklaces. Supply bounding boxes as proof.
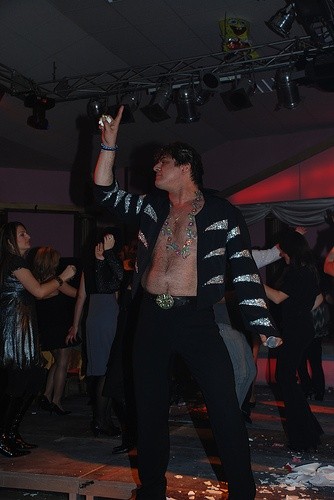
[156,191,202,258]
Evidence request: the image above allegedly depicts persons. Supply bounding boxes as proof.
[0,221,334,456]
[93,106,283,500]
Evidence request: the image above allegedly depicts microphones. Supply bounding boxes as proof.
[267,336,278,348]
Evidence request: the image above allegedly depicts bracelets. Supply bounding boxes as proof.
[100,143,118,152]
[55,277,63,287]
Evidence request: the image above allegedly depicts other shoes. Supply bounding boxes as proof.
[248,401,256,408]
[111,444,128,454]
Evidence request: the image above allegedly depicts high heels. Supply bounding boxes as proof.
[48,402,72,416]
[315,388,325,401]
[304,390,313,400]
[287,436,321,454]
[39,395,52,410]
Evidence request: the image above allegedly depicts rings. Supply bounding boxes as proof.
[109,236,112,240]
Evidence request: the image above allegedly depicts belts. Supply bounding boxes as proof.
[140,290,194,311]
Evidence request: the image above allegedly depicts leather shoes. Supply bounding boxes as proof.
[91,421,120,436]
[9,431,37,448]
[0,432,31,457]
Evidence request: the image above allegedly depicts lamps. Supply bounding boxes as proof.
[113,94,138,121]
[227,80,255,109]
[264,2,297,40]
[86,97,106,133]
[140,82,174,124]
[179,85,200,123]
[280,81,302,112]
[193,72,219,99]
[24,96,56,131]
